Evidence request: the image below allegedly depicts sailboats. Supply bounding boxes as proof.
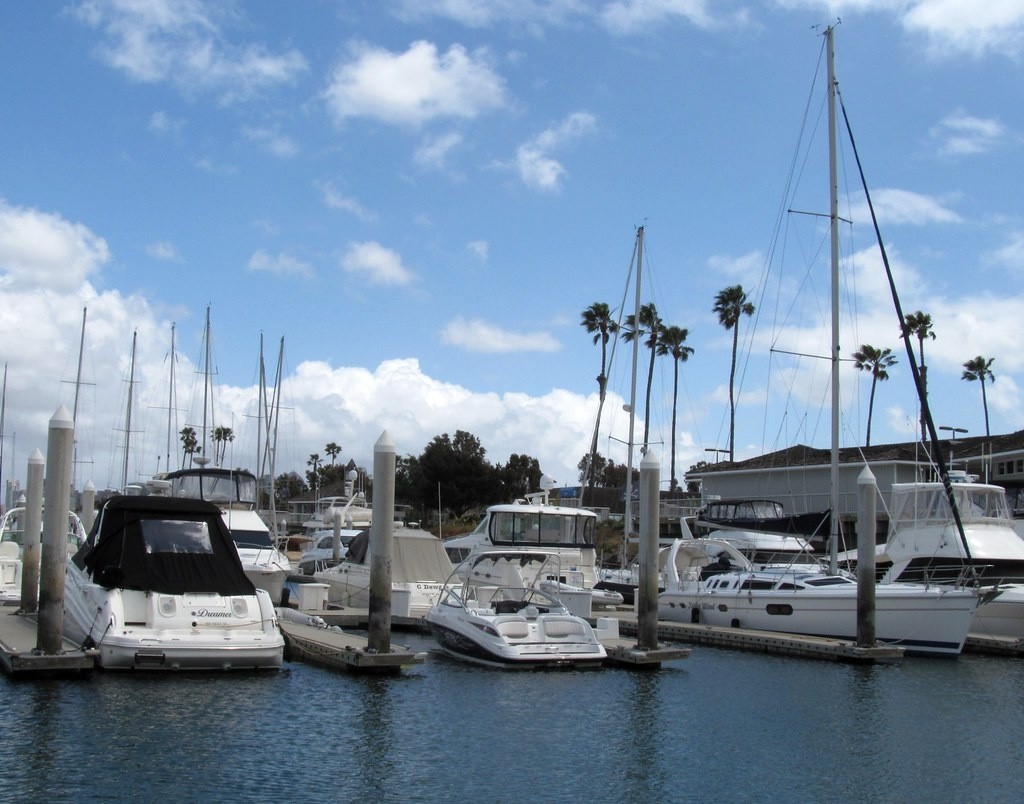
[2,9,1024,654]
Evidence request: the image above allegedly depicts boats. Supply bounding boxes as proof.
[419,549,610,673]
[60,494,285,675]
